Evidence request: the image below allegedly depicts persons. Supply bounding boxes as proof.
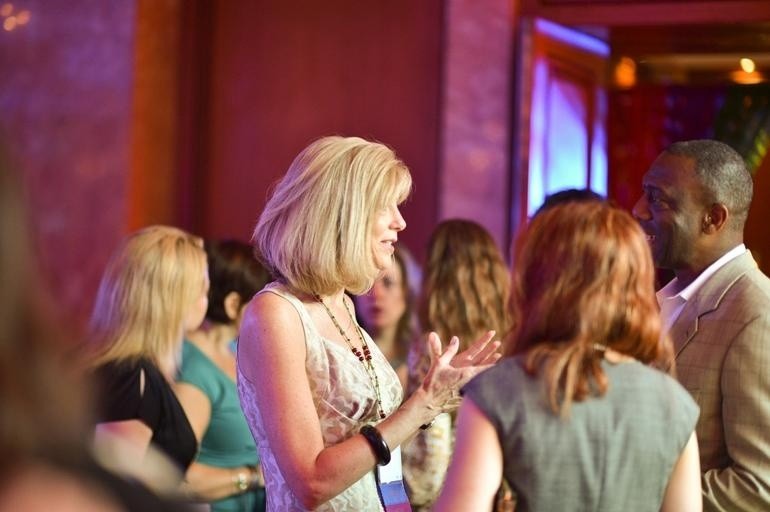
[171,240,275,510]
[436,187,702,510]
[346,241,425,403]
[76,226,266,512]
[631,140,769,511]
[401,215,513,510]
[239,136,502,510]
[0,149,126,511]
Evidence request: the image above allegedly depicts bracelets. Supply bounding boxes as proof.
[246,462,260,492]
[360,423,391,465]
[232,467,249,493]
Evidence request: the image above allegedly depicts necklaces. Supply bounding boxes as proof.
[312,291,385,418]
